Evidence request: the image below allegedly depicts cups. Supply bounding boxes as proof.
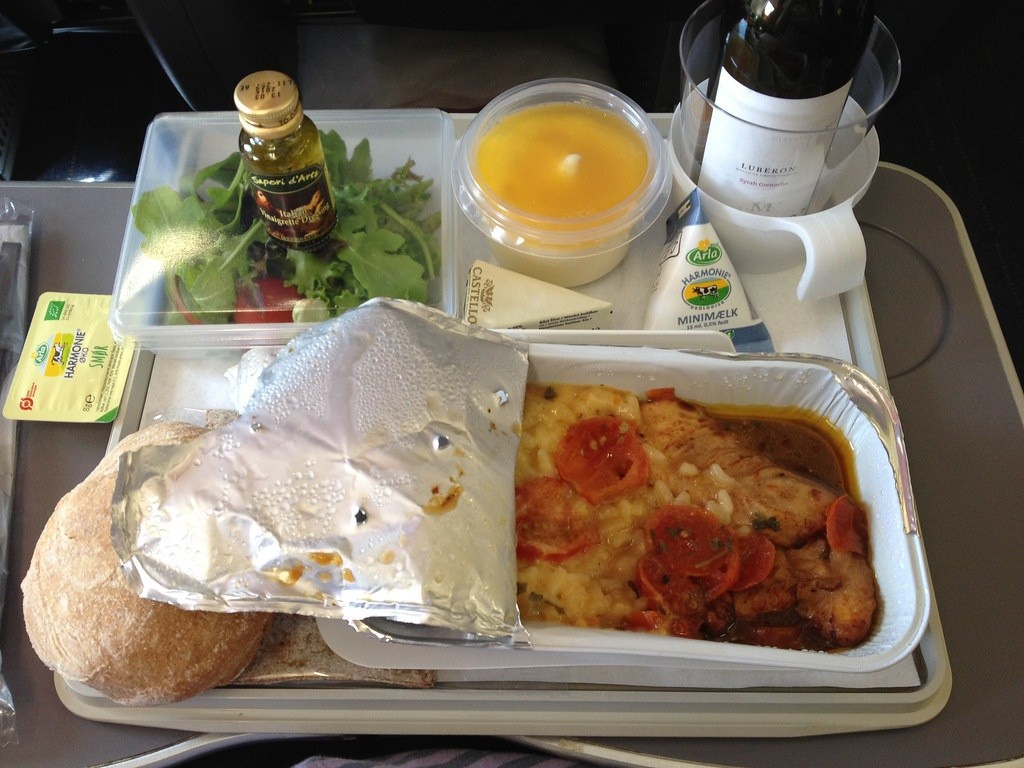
[669,78,880,301]
[679,0,901,218]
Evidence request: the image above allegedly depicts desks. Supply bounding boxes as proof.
[0,160,1024,768]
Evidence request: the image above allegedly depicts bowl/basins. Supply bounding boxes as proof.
[450,76,672,287]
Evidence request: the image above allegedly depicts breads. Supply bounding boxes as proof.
[21,419,273,708]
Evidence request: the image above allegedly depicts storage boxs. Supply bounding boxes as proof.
[314,336,935,690]
[106,110,458,360]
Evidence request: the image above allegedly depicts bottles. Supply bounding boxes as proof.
[690,0,875,218]
[233,71,338,253]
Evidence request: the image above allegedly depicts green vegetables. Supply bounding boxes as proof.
[131,129,440,323]
